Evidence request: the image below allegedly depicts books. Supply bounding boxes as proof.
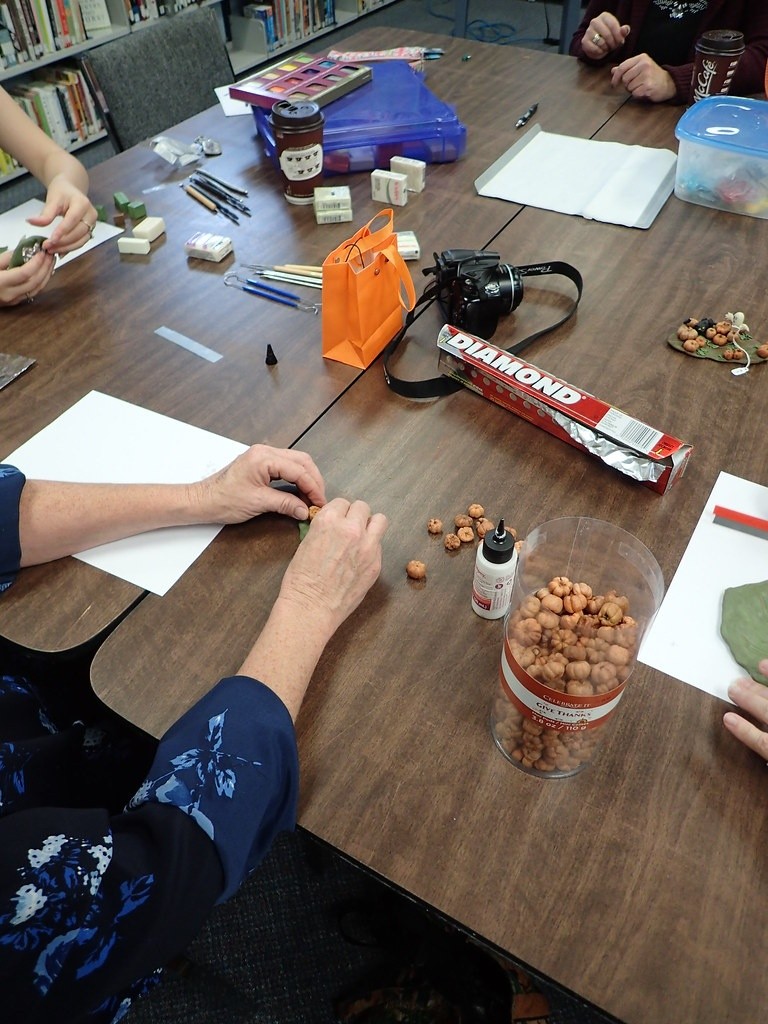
[0,0,199,177]
[244,0,384,52]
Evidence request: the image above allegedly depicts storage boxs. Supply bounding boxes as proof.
[250,60,466,179]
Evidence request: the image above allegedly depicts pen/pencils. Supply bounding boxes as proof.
[515,102,540,128]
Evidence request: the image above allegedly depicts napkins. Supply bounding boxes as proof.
[214,85,254,116]
[634,472,768,705]
[0,390,256,596]
[0,197,123,269]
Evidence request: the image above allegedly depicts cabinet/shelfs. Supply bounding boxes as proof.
[0,1,392,187]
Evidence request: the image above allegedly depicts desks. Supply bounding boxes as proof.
[0,30,768,1024]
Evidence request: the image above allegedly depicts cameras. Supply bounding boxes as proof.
[435,248,524,340]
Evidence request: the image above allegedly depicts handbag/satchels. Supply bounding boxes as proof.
[321,207,417,369]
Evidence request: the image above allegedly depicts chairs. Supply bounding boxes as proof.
[79,9,236,153]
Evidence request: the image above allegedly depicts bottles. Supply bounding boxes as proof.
[470,518,519,620]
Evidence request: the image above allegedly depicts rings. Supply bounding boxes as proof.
[80,220,95,230]
[25,292,33,304]
[592,34,602,45]
[87,232,94,240]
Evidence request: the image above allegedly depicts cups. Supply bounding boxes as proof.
[687,31,746,112]
[489,520,666,780]
[266,99,325,205]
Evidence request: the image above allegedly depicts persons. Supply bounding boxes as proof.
[569,0,768,106]
[0,84,98,306]
[723,658,768,760]
[0,444,390,1024]
